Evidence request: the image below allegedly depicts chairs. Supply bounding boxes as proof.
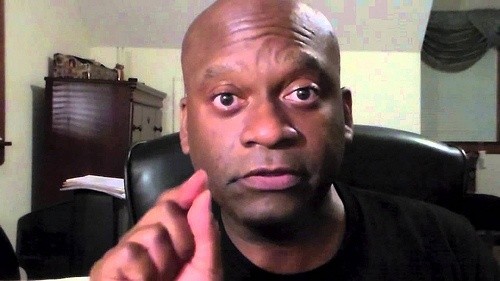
[123,122,471,224]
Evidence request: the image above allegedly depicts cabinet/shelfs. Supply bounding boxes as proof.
[45,76,170,198]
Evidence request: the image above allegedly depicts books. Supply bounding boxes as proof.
[59,174,126,199]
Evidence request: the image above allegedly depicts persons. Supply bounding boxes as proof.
[89,0,500,281]
